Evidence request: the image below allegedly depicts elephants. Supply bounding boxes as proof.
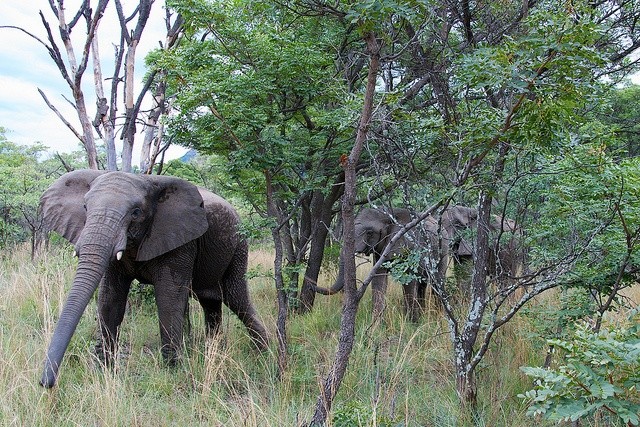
[307,205,450,326]
[37,168,269,389]
[440,205,522,307]
